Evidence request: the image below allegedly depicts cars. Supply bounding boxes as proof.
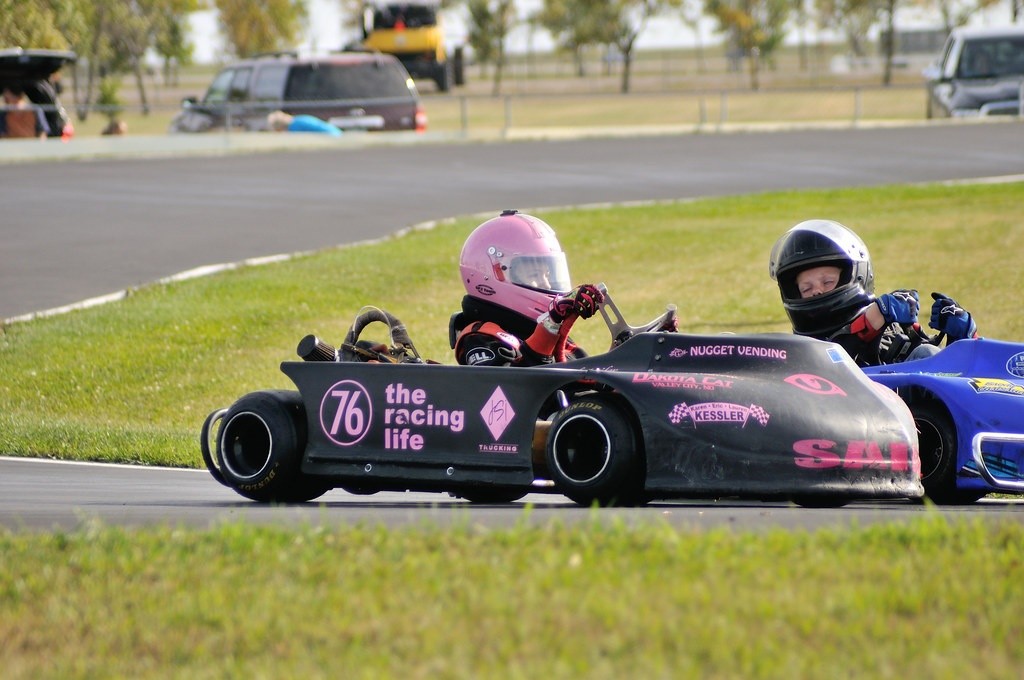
[922,24,1024,117]
[0,46,78,141]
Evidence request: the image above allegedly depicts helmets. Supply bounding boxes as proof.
[769,217,875,338]
[460,210,572,322]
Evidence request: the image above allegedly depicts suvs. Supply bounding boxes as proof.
[172,46,428,133]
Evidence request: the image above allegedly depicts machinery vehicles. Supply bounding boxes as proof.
[358,0,465,92]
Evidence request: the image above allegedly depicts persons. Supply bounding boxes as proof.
[768,219,980,367]
[450,210,678,367]
[0,62,72,139]
[266,108,344,133]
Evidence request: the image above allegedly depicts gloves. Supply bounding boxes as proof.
[540,284,603,335]
[877,287,921,326]
[929,291,978,338]
